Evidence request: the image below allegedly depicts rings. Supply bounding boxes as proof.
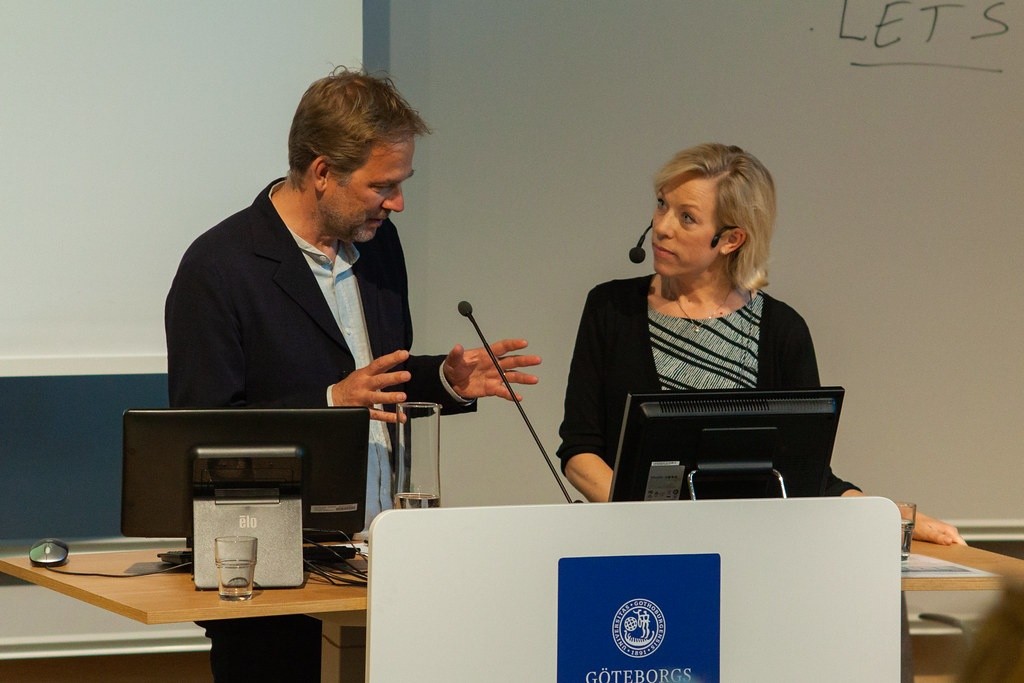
[503,371,506,377]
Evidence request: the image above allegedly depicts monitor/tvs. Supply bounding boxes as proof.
[609,385,846,504]
[123,407,371,591]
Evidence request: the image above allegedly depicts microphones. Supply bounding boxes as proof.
[630,224,653,263]
[458,300,573,504]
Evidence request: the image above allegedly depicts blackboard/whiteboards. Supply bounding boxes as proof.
[364,0,1023,544]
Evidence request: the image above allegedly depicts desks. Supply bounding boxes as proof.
[0,540,1024,682]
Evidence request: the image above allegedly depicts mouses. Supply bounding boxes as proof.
[29,538,69,568]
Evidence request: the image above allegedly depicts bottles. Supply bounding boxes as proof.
[395,402,441,509]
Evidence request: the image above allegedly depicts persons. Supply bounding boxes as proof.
[555,144,970,547]
[165,64,543,541]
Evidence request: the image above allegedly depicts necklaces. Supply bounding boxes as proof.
[669,279,733,333]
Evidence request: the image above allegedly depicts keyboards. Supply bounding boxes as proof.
[158,545,360,568]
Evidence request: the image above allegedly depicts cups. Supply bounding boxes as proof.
[897,502,917,569]
[214,536,257,601]
